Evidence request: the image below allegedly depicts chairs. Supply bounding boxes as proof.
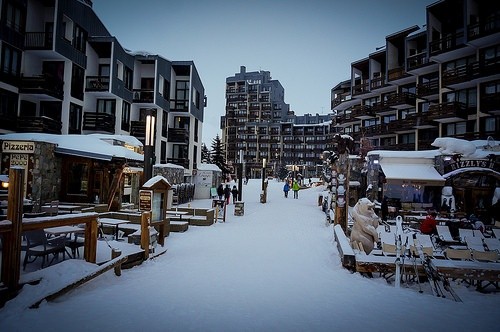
[0,201,86,271]
[379,203,500,263]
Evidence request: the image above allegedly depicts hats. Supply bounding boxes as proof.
[233,186,236,188]
[220,184,223,187]
[469,214,476,220]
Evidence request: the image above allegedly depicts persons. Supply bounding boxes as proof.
[292,180,301,199]
[231,184,239,205]
[420,212,440,234]
[221,184,232,205]
[217,184,223,200]
[283,182,290,198]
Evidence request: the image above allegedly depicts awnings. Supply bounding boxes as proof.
[380,162,446,185]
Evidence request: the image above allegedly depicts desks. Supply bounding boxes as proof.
[404,215,448,221]
[96,219,130,242]
[48,206,81,214]
[166,211,188,221]
[45,225,85,259]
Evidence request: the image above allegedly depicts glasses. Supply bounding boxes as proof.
[434,215,436,218]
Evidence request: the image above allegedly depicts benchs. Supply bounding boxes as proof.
[46,203,214,233]
